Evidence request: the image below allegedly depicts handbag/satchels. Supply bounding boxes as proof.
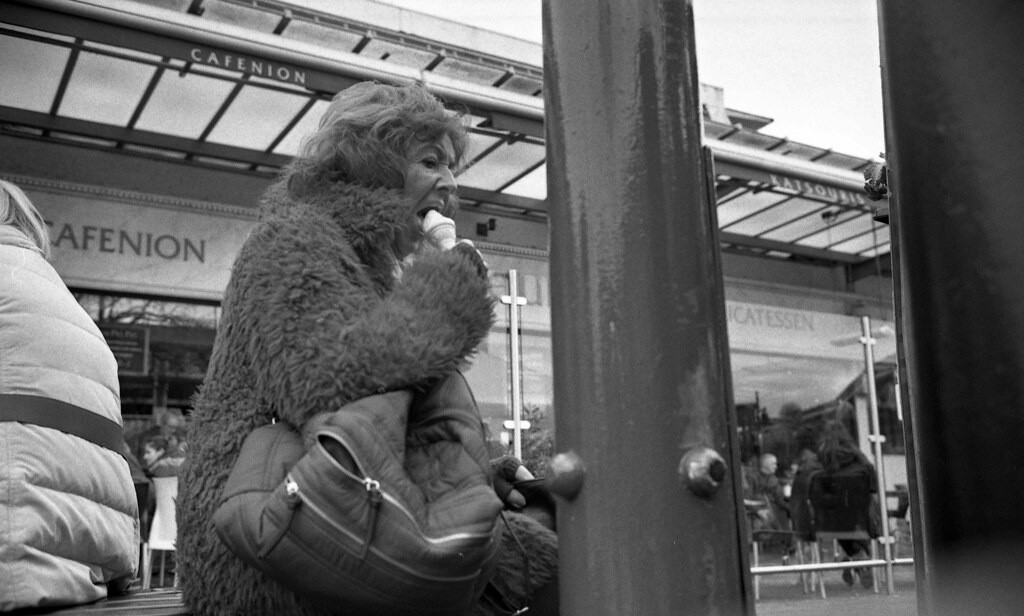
[220,363,503,616]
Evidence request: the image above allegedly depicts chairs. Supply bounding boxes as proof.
[743,470,878,601]
[135,476,180,590]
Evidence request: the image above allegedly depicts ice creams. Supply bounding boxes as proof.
[422,208,455,253]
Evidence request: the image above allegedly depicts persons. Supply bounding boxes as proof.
[124,409,190,552]
[746,453,789,565]
[175,81,558,616]
[0,179,142,616]
[790,420,877,589]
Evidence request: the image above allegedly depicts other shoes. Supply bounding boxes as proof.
[841,557,873,590]
